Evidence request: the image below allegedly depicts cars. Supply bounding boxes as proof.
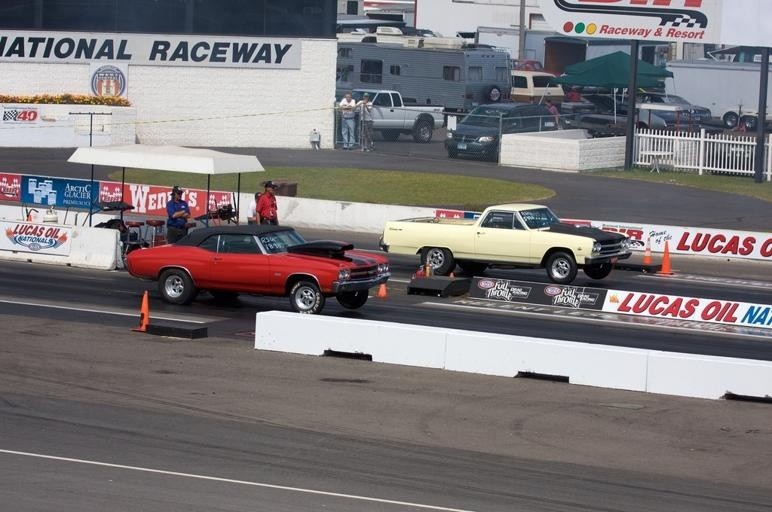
[446,59,711,160]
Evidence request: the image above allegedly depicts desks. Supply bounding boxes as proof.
[267,182,297,197]
[640,151,673,174]
[90,200,133,232]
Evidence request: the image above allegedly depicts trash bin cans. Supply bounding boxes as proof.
[261,180,298,197]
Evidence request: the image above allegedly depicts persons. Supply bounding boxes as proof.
[247,192,262,224]
[339,93,356,150]
[352,92,376,151]
[166,186,191,243]
[544,98,561,118]
[257,180,280,225]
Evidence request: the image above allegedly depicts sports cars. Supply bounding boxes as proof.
[127,225,391,314]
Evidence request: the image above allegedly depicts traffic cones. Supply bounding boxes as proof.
[130,291,150,332]
[657,241,674,274]
[643,238,652,265]
[378,285,387,298]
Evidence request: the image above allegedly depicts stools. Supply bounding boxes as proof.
[123,220,145,263]
[146,219,165,248]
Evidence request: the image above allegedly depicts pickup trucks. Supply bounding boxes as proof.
[334,88,444,143]
[379,203,632,284]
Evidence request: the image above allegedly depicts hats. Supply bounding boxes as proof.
[171,185,184,195]
[265,181,279,188]
